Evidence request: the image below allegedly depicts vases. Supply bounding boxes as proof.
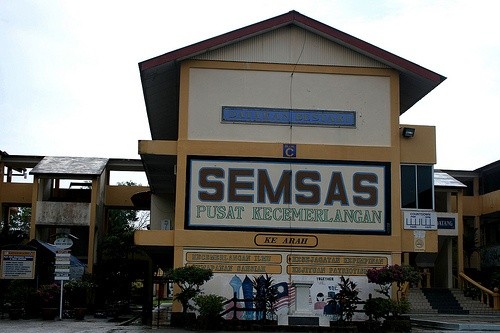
[42,307,58,321]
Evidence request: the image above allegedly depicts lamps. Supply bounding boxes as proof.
[402,127,415,137]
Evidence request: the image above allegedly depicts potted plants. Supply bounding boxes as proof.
[163,264,214,329]
[63,277,95,321]
[8,277,27,321]
[193,294,229,330]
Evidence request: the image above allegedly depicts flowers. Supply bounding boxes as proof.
[41,282,60,306]
